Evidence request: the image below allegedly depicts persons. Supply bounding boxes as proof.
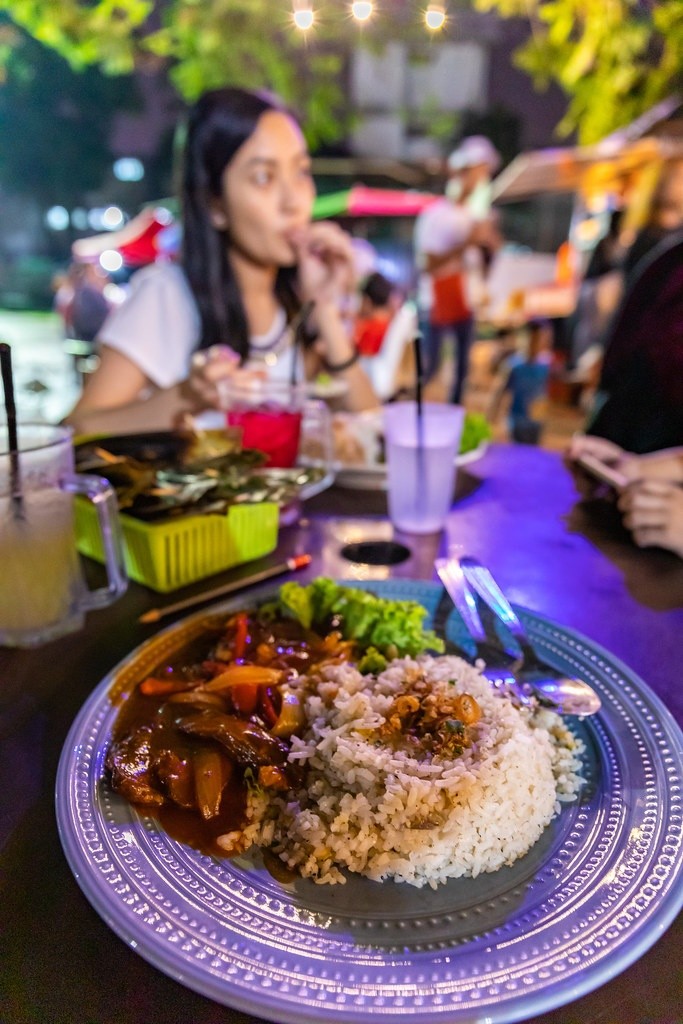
[563,211,683,562]
[483,318,566,444]
[565,204,629,409]
[66,263,115,387]
[411,136,501,404]
[56,83,388,446]
[621,197,683,277]
[353,274,399,359]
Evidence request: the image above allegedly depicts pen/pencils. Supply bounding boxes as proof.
[133,552,315,627]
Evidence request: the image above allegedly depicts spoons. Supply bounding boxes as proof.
[460,557,602,716]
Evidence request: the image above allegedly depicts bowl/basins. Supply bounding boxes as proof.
[74,494,279,595]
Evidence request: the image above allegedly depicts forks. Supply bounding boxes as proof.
[433,557,526,709]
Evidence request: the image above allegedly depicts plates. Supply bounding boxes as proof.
[56,576,682,1023]
[301,436,489,489]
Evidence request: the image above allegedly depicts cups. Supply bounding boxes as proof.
[217,379,338,527]
[0,423,131,648]
[383,402,464,535]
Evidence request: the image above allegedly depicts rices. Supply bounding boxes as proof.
[226,657,585,891]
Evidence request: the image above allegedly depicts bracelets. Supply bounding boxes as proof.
[316,345,362,372]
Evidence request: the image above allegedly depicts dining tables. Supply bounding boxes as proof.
[2,437,683,1022]
